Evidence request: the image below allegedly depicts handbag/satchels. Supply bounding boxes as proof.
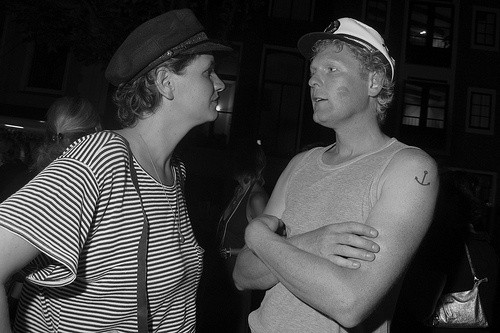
[432,278,488,328]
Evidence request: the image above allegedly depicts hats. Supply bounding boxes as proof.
[104,8,233,87]
[298,18,395,84]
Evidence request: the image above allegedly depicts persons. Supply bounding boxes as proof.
[234,18,440,333]
[208,138,270,333]
[390,140,481,333]
[23,94,103,179]
[0,8,233,333]
[0,126,19,204]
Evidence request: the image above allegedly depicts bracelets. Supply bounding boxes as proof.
[225,248,231,257]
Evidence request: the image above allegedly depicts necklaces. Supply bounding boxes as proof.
[139,136,184,242]
